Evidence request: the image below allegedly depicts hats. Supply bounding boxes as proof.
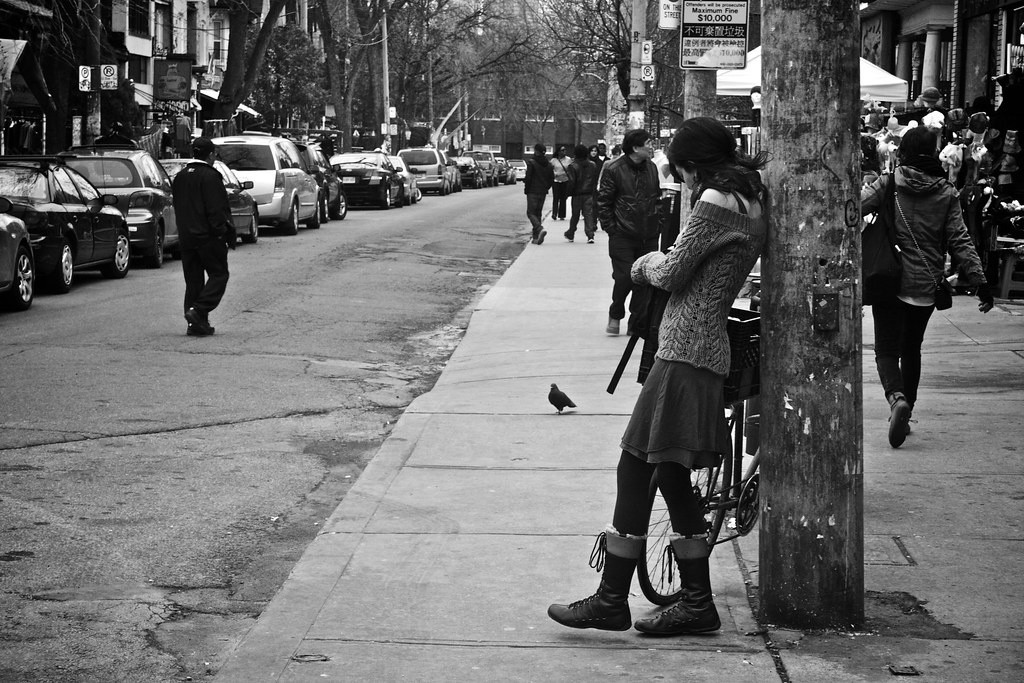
[860,109,1022,174]
[532,143,546,154]
[191,136,215,157]
[922,87,939,102]
[750,86,761,94]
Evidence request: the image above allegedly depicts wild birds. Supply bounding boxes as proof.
[548,383,578,416]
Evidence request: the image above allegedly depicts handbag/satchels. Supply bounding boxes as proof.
[626,280,670,340]
[567,180,574,197]
[936,275,954,312]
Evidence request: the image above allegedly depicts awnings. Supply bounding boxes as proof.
[199,88,260,119]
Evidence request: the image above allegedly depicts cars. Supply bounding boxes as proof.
[330,149,405,210]
[158,158,260,244]
[494,157,517,185]
[58,141,179,268]
[450,156,488,189]
[387,156,418,206]
[0,155,129,291]
[442,151,462,193]
[0,196,36,311]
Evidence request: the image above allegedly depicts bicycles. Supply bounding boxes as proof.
[637,293,763,605]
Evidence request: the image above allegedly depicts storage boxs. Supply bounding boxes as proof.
[636,304,761,395]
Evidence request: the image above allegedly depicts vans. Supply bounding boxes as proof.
[210,132,322,235]
[397,148,453,193]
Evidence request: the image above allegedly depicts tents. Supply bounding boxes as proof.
[715,43,909,112]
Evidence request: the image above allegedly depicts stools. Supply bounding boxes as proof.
[998,252,1024,299]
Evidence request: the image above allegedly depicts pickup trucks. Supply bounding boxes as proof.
[462,151,500,187]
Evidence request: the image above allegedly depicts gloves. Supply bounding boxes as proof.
[860,170,901,307]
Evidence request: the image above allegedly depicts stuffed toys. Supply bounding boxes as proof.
[877,134,898,155]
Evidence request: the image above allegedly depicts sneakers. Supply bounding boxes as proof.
[184,304,214,335]
[587,237,594,243]
[564,232,574,242]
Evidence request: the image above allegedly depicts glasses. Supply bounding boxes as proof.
[561,149,567,152]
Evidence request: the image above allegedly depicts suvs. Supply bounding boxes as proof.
[508,160,527,180]
[294,142,347,222]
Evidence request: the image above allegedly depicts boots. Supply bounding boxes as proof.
[549,525,645,632]
[635,532,721,636]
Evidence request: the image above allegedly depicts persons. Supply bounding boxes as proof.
[563,145,598,243]
[598,129,665,336]
[861,136,882,229]
[750,86,762,111]
[588,143,621,232]
[171,137,236,336]
[861,126,994,449]
[523,143,555,244]
[548,117,769,635]
[550,145,572,220]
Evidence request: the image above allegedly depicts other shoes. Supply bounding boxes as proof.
[606,317,620,334]
[561,218,564,220]
[532,229,547,245]
[888,400,912,448]
[552,214,556,220]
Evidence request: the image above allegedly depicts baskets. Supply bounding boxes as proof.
[636,310,762,404]
